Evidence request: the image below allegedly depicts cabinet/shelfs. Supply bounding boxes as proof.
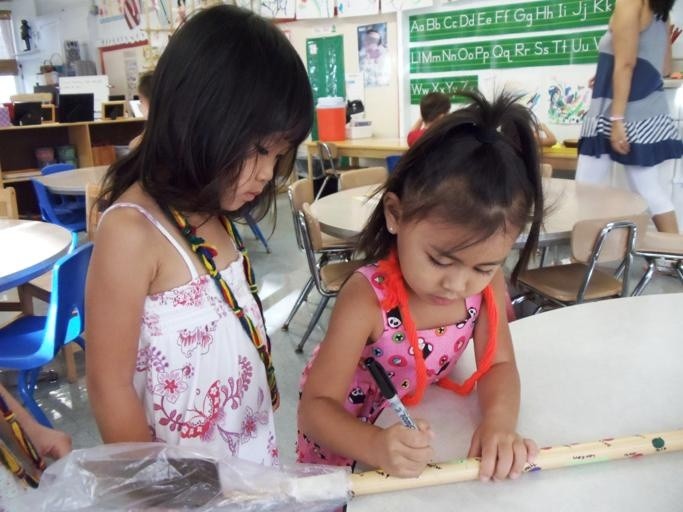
[1,116,147,219]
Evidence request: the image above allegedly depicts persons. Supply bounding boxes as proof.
[85,5,315,476]
[294,90,559,512]
[358,29,387,92]
[127,73,155,155]
[534,122,557,148]
[407,91,450,150]
[0,381,72,480]
[573,0,683,275]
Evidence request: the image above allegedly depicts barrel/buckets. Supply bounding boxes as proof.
[316,97,346,141]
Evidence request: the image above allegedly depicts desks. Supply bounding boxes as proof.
[312,178,650,299]
[342,291,683,511]
[304,137,418,183]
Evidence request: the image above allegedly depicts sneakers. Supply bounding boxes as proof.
[654,257,677,272]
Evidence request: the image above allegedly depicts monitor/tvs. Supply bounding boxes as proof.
[58,93,94,123]
[14,102,41,126]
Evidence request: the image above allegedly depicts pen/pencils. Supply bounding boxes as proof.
[364,355,421,432]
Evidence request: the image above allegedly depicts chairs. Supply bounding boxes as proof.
[615,226,682,296]
[287,178,363,304]
[1,161,118,437]
[337,166,387,189]
[282,202,373,354]
[521,163,554,271]
[509,213,651,318]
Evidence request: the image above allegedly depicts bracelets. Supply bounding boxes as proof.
[609,116,624,122]
